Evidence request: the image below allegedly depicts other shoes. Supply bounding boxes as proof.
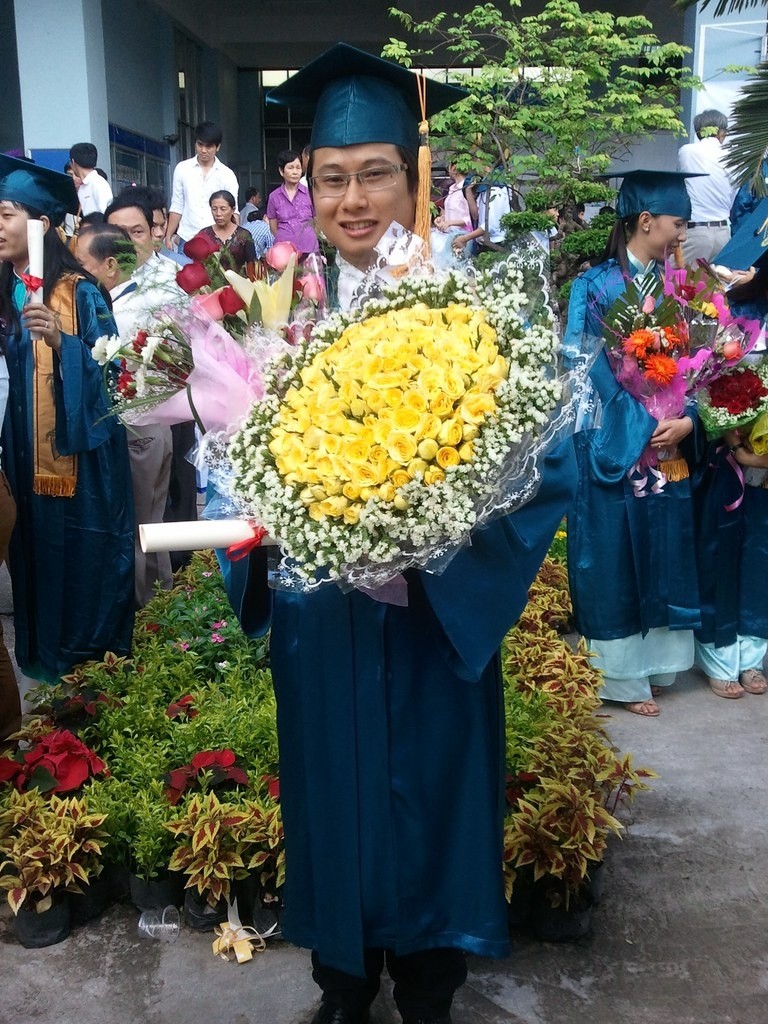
[311,993,371,1024]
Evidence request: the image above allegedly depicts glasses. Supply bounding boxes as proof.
[306,163,409,198]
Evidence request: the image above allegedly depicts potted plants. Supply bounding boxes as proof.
[0,517,660,950]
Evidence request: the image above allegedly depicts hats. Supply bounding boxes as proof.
[0,151,84,256]
[265,41,472,279]
[593,168,711,268]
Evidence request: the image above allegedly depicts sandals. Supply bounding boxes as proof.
[741,669,768,694]
[651,685,662,697]
[702,671,745,699]
[622,699,661,717]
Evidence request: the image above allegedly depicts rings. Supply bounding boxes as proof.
[658,443,661,449]
[45,321,48,327]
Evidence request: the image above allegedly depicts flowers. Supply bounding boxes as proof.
[88,212,599,673]
[600,243,768,456]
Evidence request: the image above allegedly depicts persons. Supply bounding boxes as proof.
[562,147,768,717]
[0,121,318,756]
[669,110,741,272]
[432,139,510,259]
[213,43,579,1024]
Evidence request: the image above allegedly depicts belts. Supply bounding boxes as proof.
[687,220,727,229]
